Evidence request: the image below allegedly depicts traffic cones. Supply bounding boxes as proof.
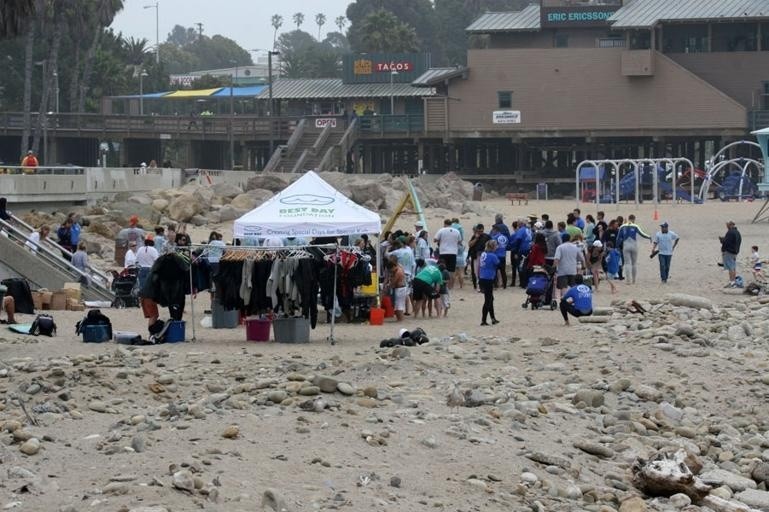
[653,209,658,220]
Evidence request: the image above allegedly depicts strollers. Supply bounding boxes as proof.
[522,262,557,311]
[107,264,143,308]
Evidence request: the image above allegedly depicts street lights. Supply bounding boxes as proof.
[390,72,398,114]
[227,73,236,169]
[138,69,149,114]
[267,50,280,157]
[35,59,49,171]
[142,2,160,64]
[52,72,60,128]
[251,47,268,63]
[228,58,238,83]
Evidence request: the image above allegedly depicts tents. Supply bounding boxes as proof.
[234,171,381,308]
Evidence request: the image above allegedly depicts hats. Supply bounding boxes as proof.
[527,212,539,219]
[414,221,423,227]
[659,222,668,227]
[129,215,136,226]
[592,240,602,248]
[395,235,406,243]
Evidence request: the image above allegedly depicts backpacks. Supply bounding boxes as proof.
[28,315,56,337]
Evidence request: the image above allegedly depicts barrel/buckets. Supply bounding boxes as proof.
[370,308,385,325]
[381,295,394,318]
[244,318,271,341]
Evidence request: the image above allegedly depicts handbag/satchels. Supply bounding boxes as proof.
[81,324,108,343]
[526,273,544,297]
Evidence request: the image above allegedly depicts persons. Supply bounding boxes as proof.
[343,106,370,128]
[189,106,199,131]
[21,150,38,176]
[0,283,19,324]
[24,224,49,257]
[58,214,232,332]
[653,222,680,283]
[751,246,769,284]
[232,221,450,322]
[718,220,742,289]
[434,209,652,326]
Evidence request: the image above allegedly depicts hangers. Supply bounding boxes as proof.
[168,244,371,264]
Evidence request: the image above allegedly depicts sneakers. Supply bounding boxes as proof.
[725,283,736,288]
